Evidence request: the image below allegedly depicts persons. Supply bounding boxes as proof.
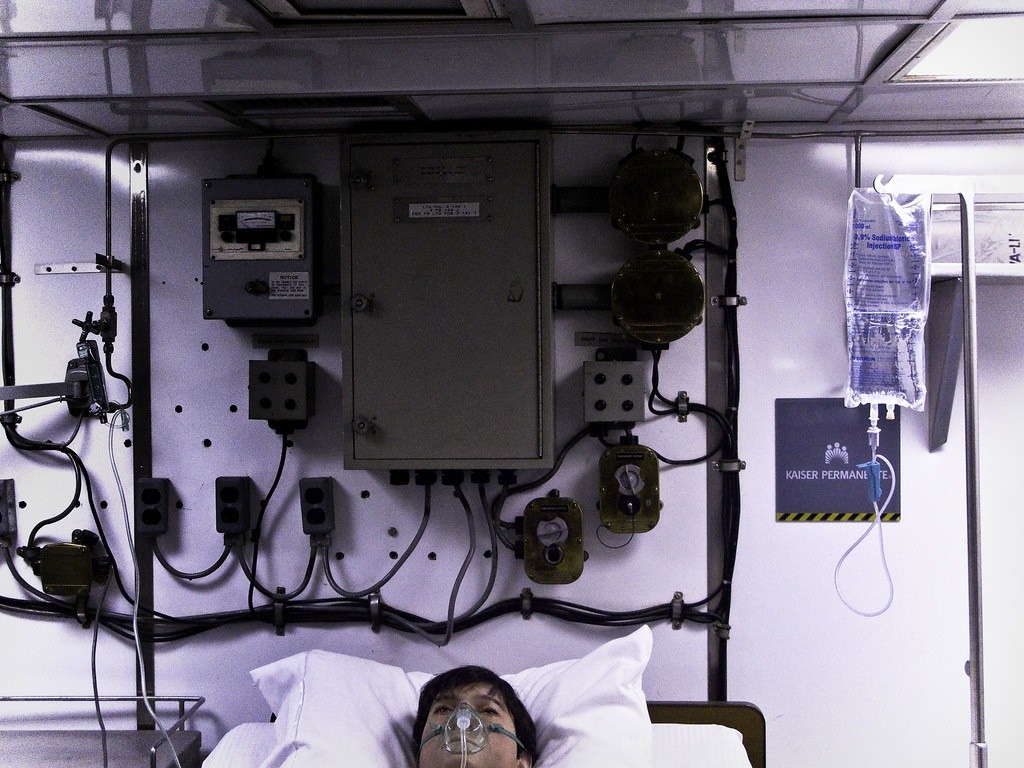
[411,664,539,768]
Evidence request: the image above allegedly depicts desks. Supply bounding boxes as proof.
[0,695,207,768]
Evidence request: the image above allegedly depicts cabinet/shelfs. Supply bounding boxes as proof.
[337,124,556,474]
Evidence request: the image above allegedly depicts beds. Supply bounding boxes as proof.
[202,697,766,768]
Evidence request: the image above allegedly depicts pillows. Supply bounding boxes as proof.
[249,625,655,768]
